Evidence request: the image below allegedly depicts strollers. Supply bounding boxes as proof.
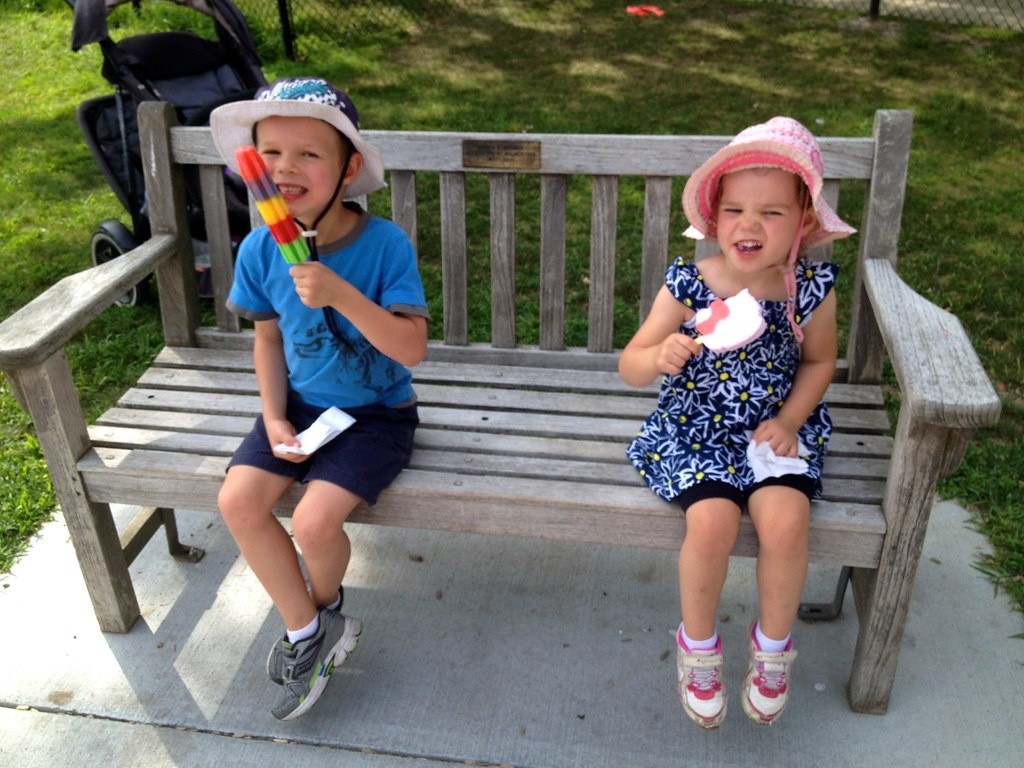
[65,0,270,309]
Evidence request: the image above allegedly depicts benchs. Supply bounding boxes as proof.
[0,102,1003,716]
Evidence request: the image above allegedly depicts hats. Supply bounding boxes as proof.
[209,78,388,200]
[681,116,857,257]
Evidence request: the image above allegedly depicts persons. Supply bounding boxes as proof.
[619,116,858,730]
[210,78,430,721]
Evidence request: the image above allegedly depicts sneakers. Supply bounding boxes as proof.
[264,584,345,687]
[270,609,363,721]
[676,619,728,729]
[740,614,793,725]
